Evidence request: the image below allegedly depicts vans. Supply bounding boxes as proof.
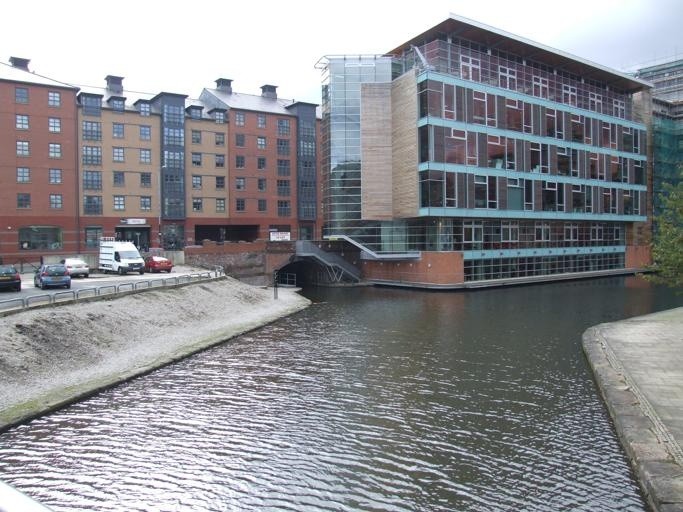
[98,240,145,275]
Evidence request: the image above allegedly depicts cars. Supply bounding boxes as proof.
[34,263,71,290]
[0,265,22,292]
[143,256,172,273]
[60,258,89,278]
